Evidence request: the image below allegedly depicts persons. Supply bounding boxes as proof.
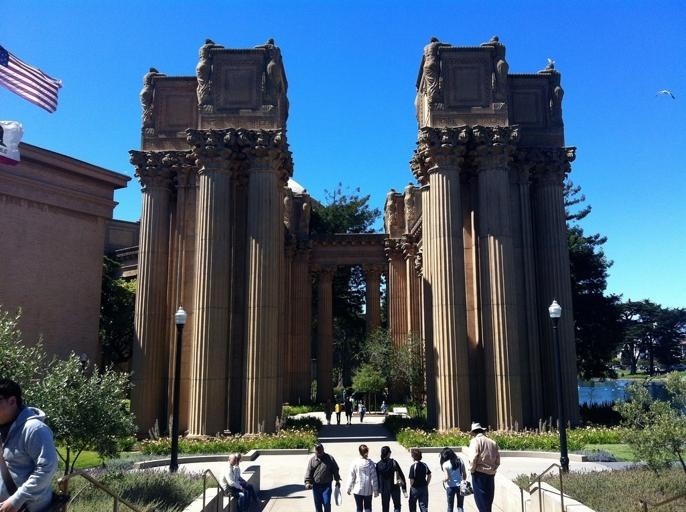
[283,188,295,234]
[140,68,167,129]
[481,36,509,103]
[403,183,420,221]
[196,39,224,106]
[0,378,58,512]
[301,193,311,235]
[439,447,466,512]
[375,446,406,512]
[304,443,342,512]
[422,37,451,104]
[324,399,389,426]
[408,449,431,512]
[387,189,402,226]
[538,57,565,131]
[347,445,378,512]
[468,422,500,512]
[222,454,249,512]
[254,39,281,106]
[233,452,262,507]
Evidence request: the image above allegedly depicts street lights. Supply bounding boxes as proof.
[169,303,190,473]
[546,300,574,471]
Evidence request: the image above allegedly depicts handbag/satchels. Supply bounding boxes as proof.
[391,458,404,486]
[333,484,342,507]
[458,479,474,496]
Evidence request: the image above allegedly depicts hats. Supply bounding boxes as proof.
[466,422,489,434]
[314,442,324,449]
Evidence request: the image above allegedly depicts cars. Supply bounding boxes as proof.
[607,361,686,379]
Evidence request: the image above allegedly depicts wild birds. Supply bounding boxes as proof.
[654,90,675,100]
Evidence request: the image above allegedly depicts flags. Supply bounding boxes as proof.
[0,44,63,113]
[0,120,24,162]
